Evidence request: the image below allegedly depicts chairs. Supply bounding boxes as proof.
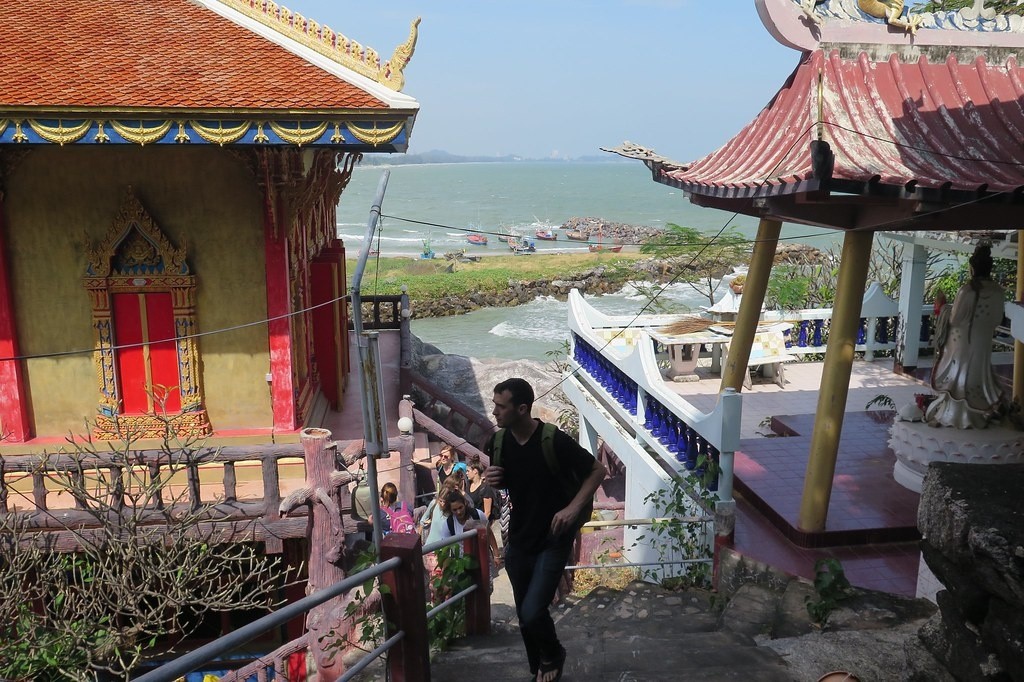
[742,330,794,390]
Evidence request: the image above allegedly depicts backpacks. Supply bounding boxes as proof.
[382,501,416,534]
[480,485,502,520]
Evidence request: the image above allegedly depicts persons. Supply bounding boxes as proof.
[923,246,1009,430]
[422,486,452,547]
[439,491,502,595]
[465,459,494,528]
[367,482,414,535]
[444,476,474,508]
[410,444,468,488]
[486,377,608,682]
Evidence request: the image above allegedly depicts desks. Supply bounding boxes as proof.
[643,324,730,382]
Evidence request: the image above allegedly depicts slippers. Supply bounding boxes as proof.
[535,648,567,682]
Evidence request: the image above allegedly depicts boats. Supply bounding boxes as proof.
[535,229,557,240]
[422,250,435,259]
[589,244,624,253]
[465,234,487,245]
[508,236,536,251]
[565,231,590,241]
[498,235,509,242]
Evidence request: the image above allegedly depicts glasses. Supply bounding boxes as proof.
[438,453,452,460]
